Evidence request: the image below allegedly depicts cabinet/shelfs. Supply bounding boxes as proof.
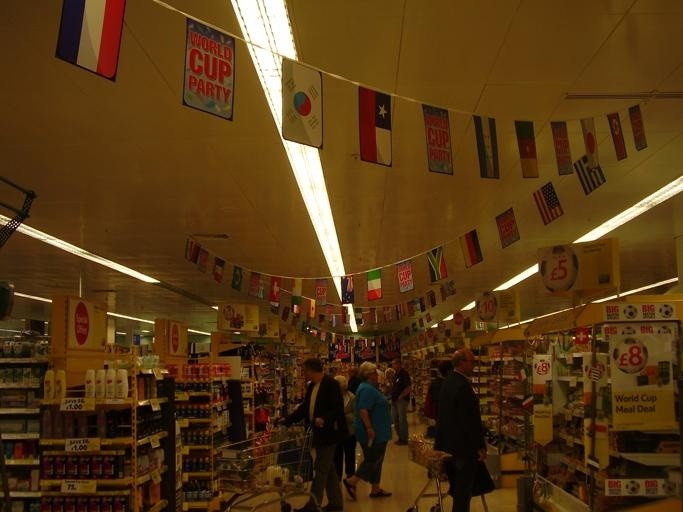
[0,354,53,511]
[480,355,526,473]
[522,337,682,511]
[36,397,169,512]
[175,383,232,511]
[213,331,273,491]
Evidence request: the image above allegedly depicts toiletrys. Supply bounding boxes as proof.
[42,368,130,399]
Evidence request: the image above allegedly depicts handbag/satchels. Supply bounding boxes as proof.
[445,459,494,496]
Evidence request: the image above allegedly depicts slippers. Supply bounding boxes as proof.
[370,488,392,496]
[344,478,357,499]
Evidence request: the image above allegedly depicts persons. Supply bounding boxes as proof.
[279,350,496,511]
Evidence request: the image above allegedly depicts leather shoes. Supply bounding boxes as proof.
[294,501,319,511]
[322,501,344,511]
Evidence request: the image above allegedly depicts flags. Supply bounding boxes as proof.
[396,260,415,293]
[183,237,225,284]
[366,269,383,301]
[280,58,323,149]
[627,104,647,151]
[421,104,454,176]
[459,229,483,269]
[495,207,521,249]
[515,120,539,178]
[439,281,457,302]
[358,86,392,168]
[607,113,628,161]
[549,121,574,176]
[472,114,499,180]
[574,153,606,197]
[182,17,235,122]
[54,1,127,83]
[427,247,448,283]
[341,276,355,304]
[532,182,564,227]
[335,290,437,350]
[232,266,336,345]
[580,118,600,168]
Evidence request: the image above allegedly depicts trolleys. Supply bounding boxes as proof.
[214,419,328,511]
[404,431,491,512]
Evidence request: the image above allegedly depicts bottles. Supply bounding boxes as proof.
[532,332,608,352]
[0,335,252,511]
[472,344,524,452]
[576,481,586,504]
